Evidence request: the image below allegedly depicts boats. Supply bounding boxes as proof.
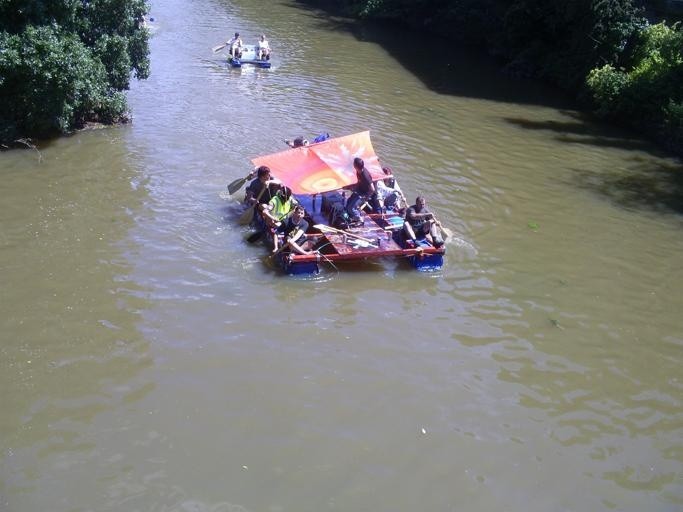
[227,45,271,68]
[243,127,443,275]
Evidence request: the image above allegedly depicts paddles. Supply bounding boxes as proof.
[238,187,266,225]
[313,223,372,243]
[212,38,238,54]
[247,208,293,244]
[228,169,257,195]
[265,244,288,268]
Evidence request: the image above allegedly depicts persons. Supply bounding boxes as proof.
[227,32,243,60]
[344,157,374,227]
[262,185,298,235]
[402,196,444,248]
[292,135,308,147]
[375,166,407,214]
[269,207,319,259]
[257,35,270,61]
[245,165,282,212]
